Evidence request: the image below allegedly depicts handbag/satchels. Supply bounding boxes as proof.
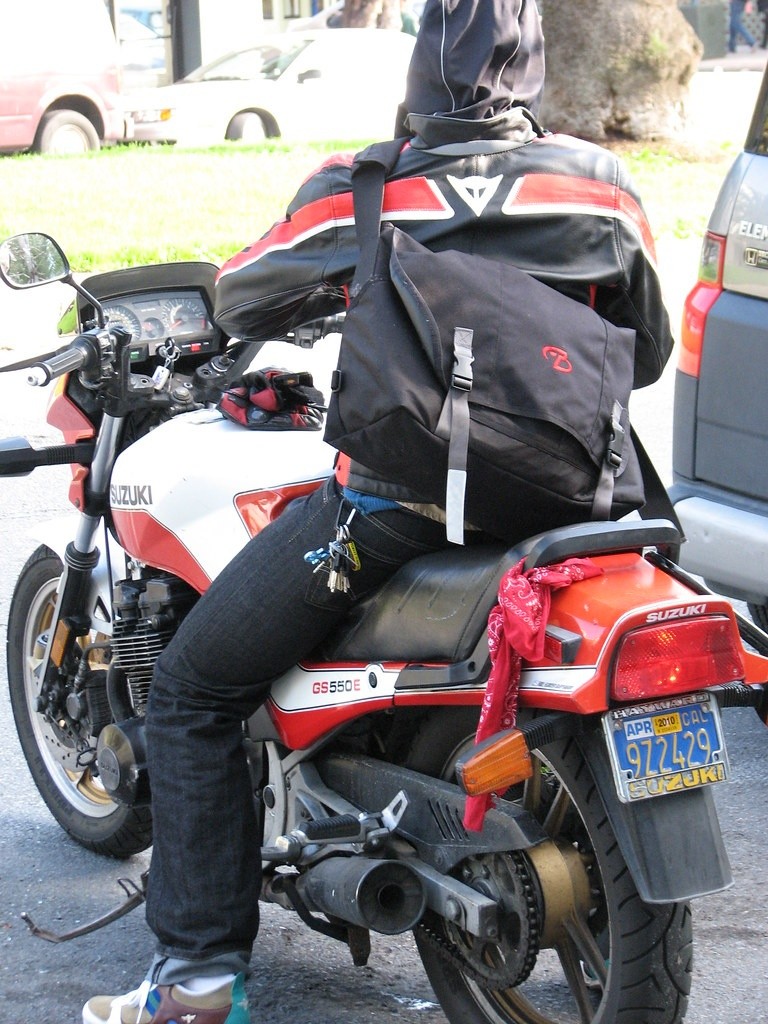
[323,221,639,544]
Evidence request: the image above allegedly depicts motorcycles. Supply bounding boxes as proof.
[0,232,768,1023]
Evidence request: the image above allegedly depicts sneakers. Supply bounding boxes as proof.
[83,971,252,1024]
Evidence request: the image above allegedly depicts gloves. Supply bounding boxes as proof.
[217,367,328,431]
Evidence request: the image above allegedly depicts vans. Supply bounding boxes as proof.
[0,0,134,155]
[666,58,767,633]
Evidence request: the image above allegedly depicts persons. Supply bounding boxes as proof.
[80,0,673,1024]
[758,0,768,49]
[729,0,757,52]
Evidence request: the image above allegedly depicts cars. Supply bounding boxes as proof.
[117,28,417,145]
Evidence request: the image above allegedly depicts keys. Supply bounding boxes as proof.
[313,544,353,593]
